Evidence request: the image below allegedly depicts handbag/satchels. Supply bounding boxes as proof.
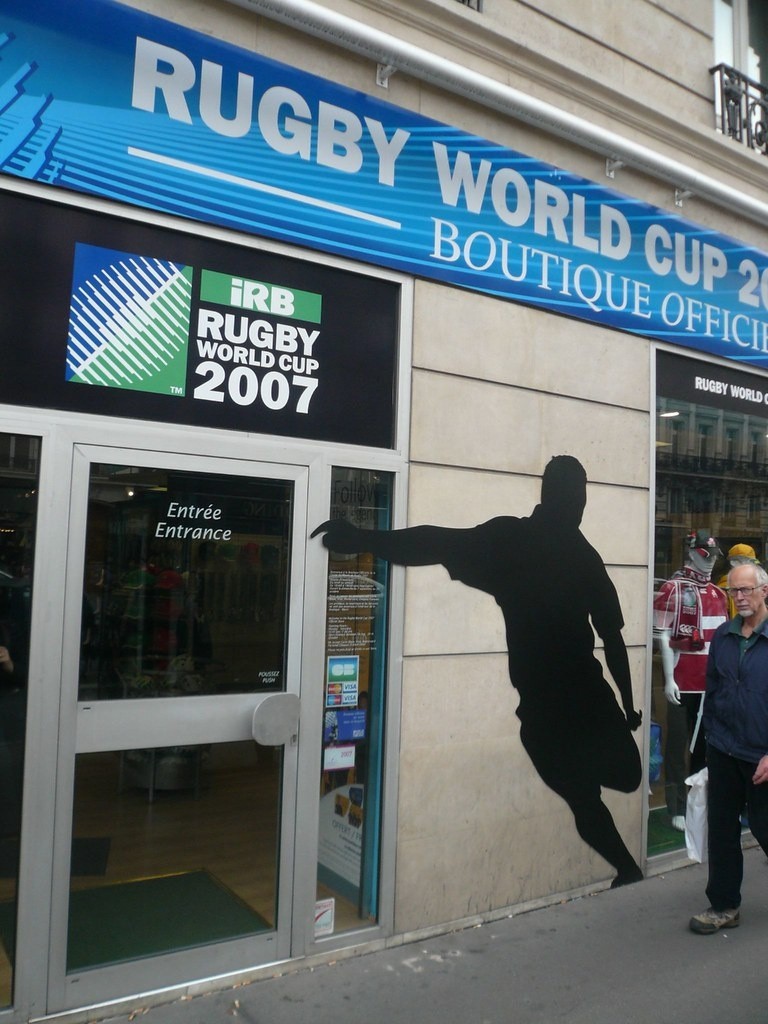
[684,767,708,863]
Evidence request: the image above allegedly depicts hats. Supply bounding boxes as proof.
[123,626,177,648]
[726,544,760,564]
[685,530,724,558]
[112,566,184,593]
[124,598,181,619]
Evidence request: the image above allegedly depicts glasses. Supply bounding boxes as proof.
[727,583,766,597]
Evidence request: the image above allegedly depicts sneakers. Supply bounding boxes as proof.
[689,907,740,935]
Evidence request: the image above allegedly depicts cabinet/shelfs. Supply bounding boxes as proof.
[118,672,210,806]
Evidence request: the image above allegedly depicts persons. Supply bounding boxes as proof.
[716,543,762,621]
[657,530,727,829]
[687,561,768,934]
